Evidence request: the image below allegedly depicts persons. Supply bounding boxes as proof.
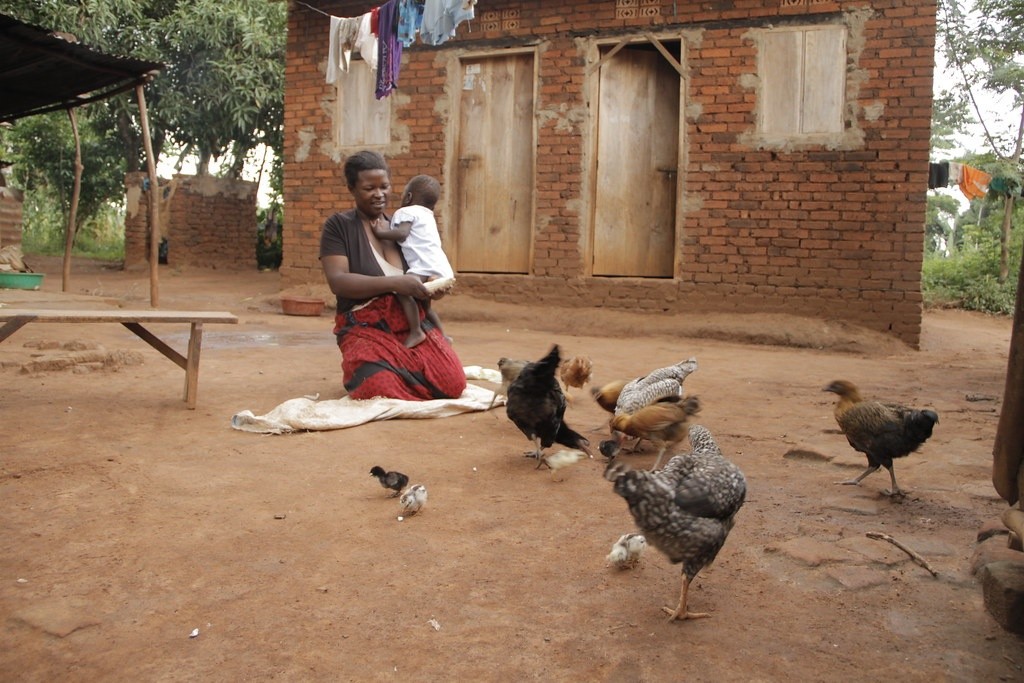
[368,174,455,352]
[319,151,469,402]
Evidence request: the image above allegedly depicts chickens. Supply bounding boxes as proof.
[821,379,939,498]
[497,343,702,484]
[369,466,409,498]
[604,424,747,622]
[399,484,427,517]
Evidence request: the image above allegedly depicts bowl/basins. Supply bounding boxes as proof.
[279,297,325,317]
[0,272,44,290]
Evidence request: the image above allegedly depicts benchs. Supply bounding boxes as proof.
[0,308,238,411]
